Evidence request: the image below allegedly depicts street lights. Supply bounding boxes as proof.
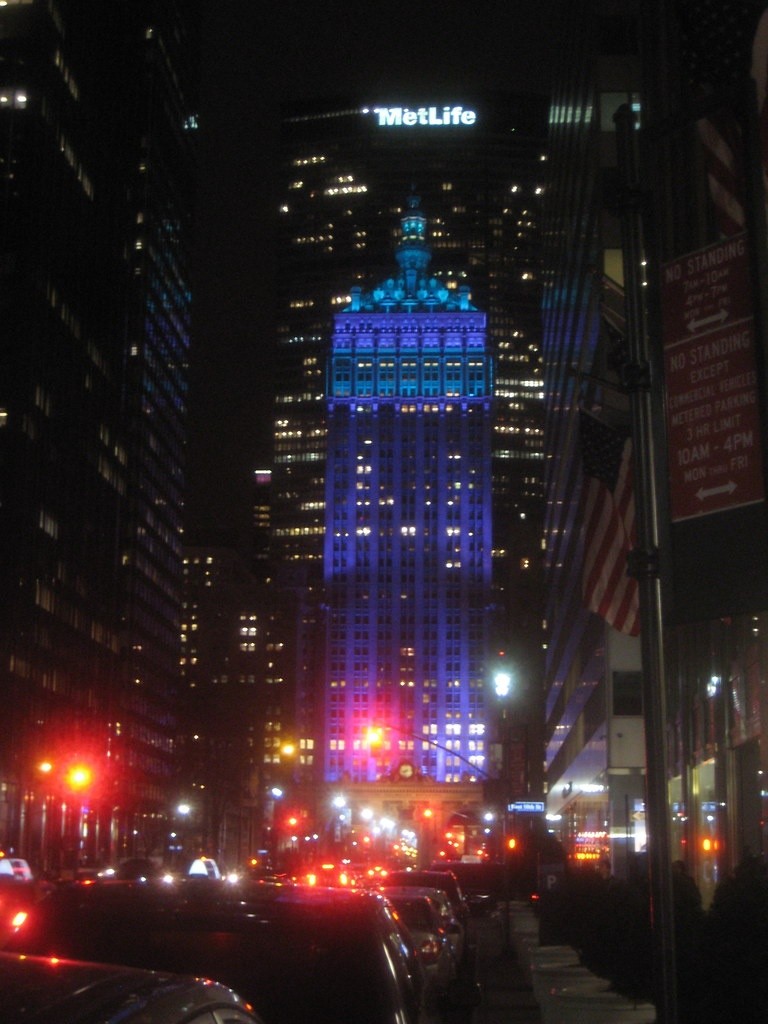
[364,722,514,935]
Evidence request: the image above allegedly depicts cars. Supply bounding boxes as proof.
[1,858,499,1024]
